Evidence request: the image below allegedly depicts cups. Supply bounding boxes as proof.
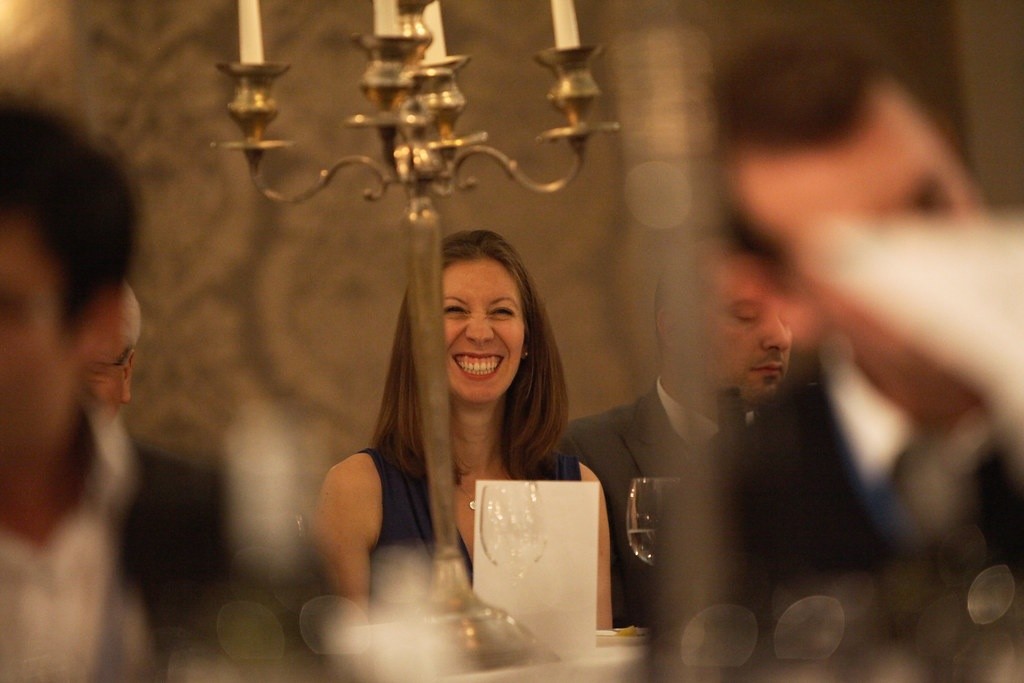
[627,473,665,564]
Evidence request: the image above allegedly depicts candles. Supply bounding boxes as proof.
[374,0,400,37]
[418,0,446,63]
[237,0,269,68]
[550,0,580,50]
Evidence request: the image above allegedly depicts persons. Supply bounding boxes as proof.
[313,228,618,681]
[694,44,1024,679]
[2,87,167,683]
[78,279,226,660]
[533,228,808,639]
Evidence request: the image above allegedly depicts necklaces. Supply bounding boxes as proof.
[445,458,510,510]
